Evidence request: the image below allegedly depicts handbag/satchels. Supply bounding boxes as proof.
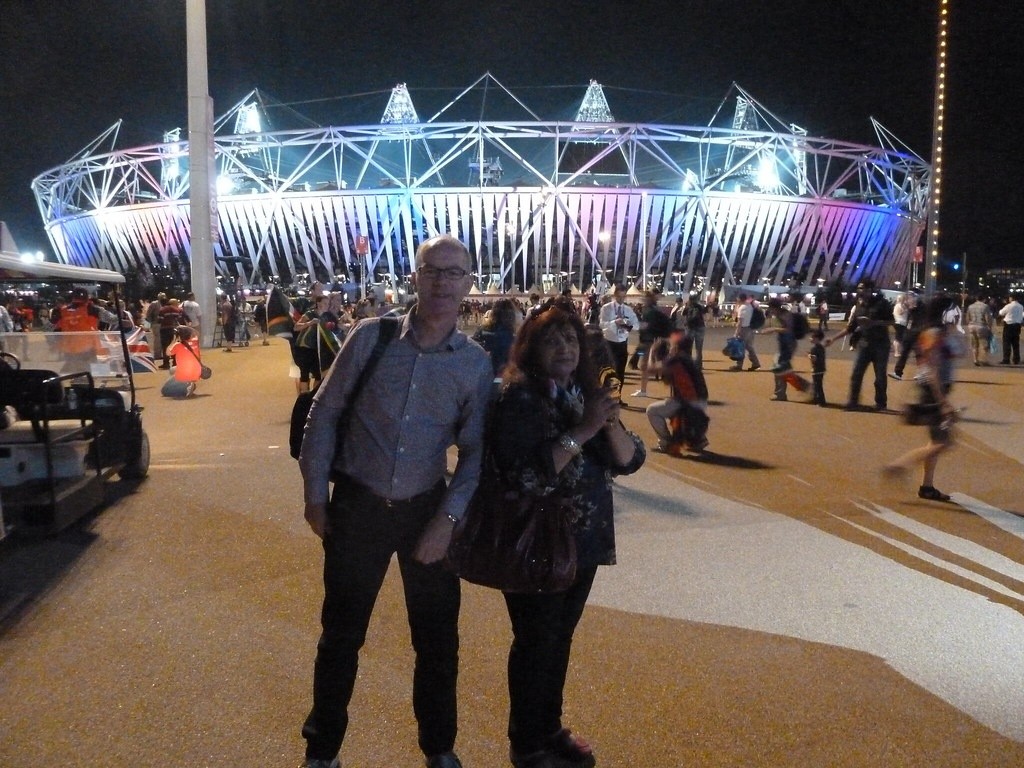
[901,401,940,425]
[200,366,211,380]
[288,313,397,459]
[722,345,728,356]
[749,301,765,329]
[792,312,809,340]
[727,335,745,361]
[443,383,578,593]
[671,305,682,327]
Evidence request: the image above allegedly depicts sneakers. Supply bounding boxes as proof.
[185,382,196,399]
[631,389,647,397]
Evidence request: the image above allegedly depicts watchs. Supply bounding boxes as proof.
[445,512,460,525]
[559,432,581,455]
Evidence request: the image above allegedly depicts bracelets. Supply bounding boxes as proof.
[604,420,621,430]
[567,431,583,453]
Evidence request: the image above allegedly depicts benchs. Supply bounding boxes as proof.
[0,372,93,448]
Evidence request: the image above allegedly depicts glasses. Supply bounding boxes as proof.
[531,299,574,315]
[414,266,472,280]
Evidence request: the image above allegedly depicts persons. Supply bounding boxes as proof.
[884,296,965,502]
[646,338,711,453]
[301,234,496,768]
[0,273,1024,422]
[484,301,647,768]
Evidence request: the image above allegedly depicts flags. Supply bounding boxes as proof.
[95,327,157,376]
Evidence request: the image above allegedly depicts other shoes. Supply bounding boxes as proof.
[868,403,887,411]
[509,743,557,768]
[888,372,901,380]
[158,364,169,369]
[423,752,462,768]
[296,755,339,768]
[729,366,742,371]
[619,400,628,408]
[974,362,980,367]
[748,365,760,371]
[770,394,788,401]
[687,438,709,450]
[656,437,670,452]
[850,346,853,351]
[918,485,951,501]
[999,360,1010,364]
[552,726,593,760]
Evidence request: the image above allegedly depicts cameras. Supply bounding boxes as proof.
[174,328,179,336]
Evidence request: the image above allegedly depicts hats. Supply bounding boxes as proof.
[650,288,661,295]
